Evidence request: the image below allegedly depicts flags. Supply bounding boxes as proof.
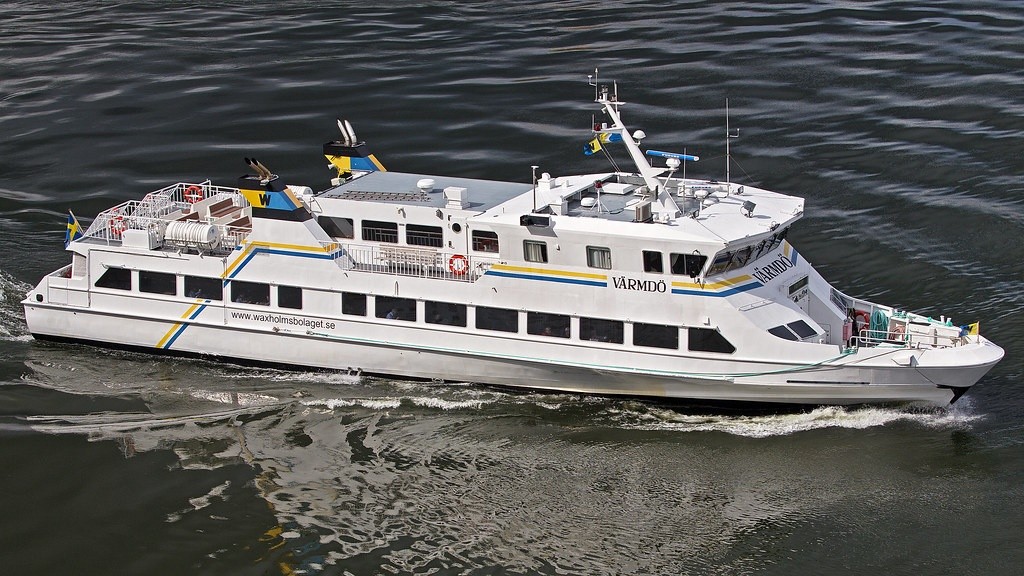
[583,138,601,156]
[64,214,83,249]
[598,132,624,145]
[959,323,977,336]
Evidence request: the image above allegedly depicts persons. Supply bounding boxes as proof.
[183,281,303,317]
[375,293,628,348]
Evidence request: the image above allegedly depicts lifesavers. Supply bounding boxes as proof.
[108,207,126,234]
[854,310,870,332]
[184,185,204,204]
[449,254,469,275]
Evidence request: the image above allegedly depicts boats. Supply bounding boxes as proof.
[21,63,1007,410]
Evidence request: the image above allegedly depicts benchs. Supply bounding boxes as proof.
[175,211,200,223]
[206,198,243,218]
[226,215,252,237]
